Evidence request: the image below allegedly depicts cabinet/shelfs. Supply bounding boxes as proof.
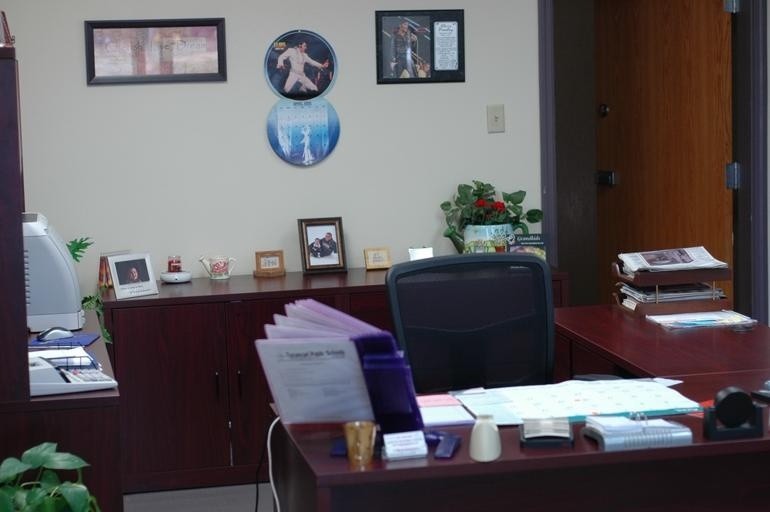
[349,286,568,388]
[105,288,348,495]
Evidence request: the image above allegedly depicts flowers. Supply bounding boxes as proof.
[440,184,543,226]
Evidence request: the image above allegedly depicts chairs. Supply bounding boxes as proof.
[383,258,558,385]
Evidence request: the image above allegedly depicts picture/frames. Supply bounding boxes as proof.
[84,17,227,86]
[252,247,285,282]
[357,244,390,268]
[105,249,158,300]
[375,8,465,86]
[298,216,346,275]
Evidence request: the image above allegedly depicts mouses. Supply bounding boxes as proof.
[37,326,73,341]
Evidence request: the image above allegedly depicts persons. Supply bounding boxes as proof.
[120,264,143,285]
[315,56,334,93]
[308,232,338,258]
[390,62,431,78]
[390,19,420,79]
[275,39,330,95]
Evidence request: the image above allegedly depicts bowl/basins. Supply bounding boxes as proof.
[160,271,192,283]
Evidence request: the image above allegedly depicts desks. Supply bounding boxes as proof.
[31,311,126,512]
[553,297,770,376]
[266,370,770,512]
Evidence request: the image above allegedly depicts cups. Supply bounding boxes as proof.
[344,421,377,466]
[469,414,502,462]
[167,255,181,272]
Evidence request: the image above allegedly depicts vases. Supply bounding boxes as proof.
[461,227,518,254]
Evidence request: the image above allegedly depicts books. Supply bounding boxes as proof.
[254,298,425,427]
[26,333,101,348]
[614,246,759,311]
